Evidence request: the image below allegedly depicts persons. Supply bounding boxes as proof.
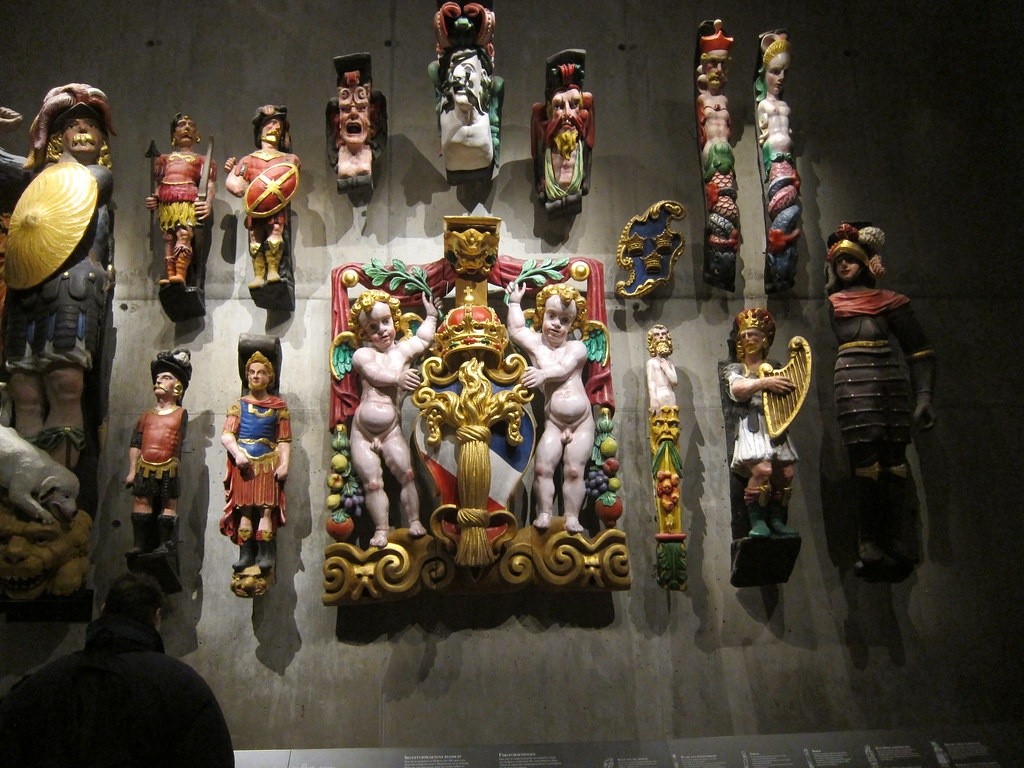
[146,113,217,287]
[725,309,798,538]
[219,350,293,571]
[647,324,678,412]
[825,222,935,563]
[5,83,117,468]
[543,61,595,200]
[125,348,192,556]
[503,280,595,534]
[697,19,732,171]
[349,289,443,547]
[224,105,301,288]
[338,69,376,176]
[436,45,493,171]
[756,33,795,159]
